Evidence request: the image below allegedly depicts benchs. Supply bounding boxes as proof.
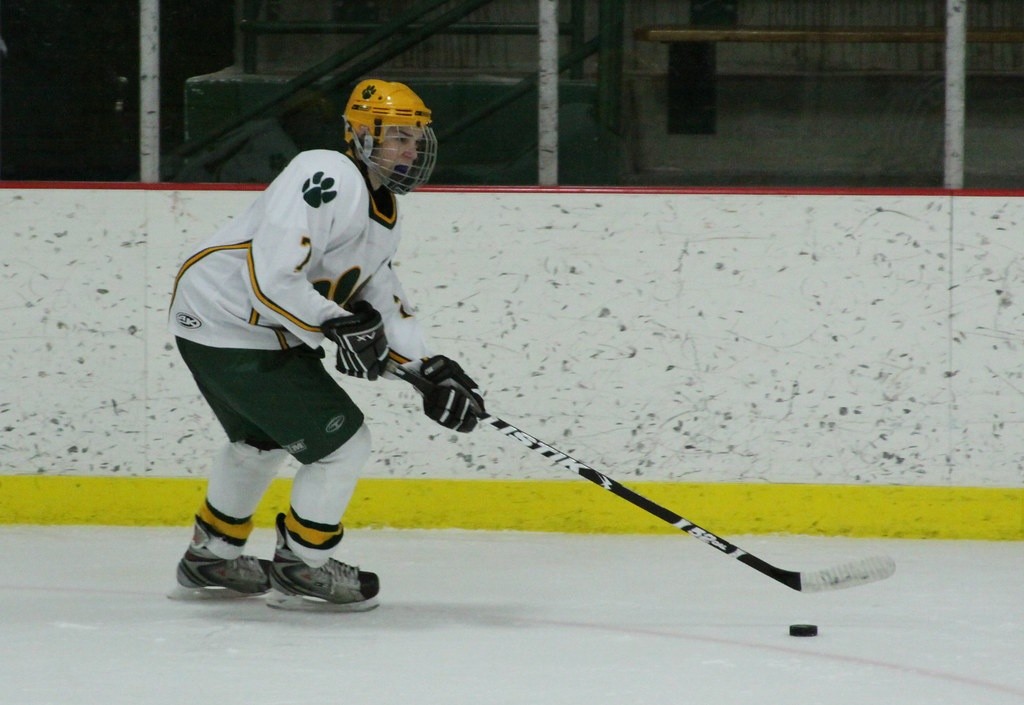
[644,0,1024,135]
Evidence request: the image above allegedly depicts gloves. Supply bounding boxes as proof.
[320,299,389,381]
[414,356,483,433]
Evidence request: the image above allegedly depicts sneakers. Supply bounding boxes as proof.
[166,514,272,601]
[265,512,380,612]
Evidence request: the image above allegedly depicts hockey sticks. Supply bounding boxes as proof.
[377,358,895,598]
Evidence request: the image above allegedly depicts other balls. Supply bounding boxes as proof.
[789,624,819,638]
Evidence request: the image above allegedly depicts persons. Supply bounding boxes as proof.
[167,79,486,613]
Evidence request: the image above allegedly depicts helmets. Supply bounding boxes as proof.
[343,80,431,146]
